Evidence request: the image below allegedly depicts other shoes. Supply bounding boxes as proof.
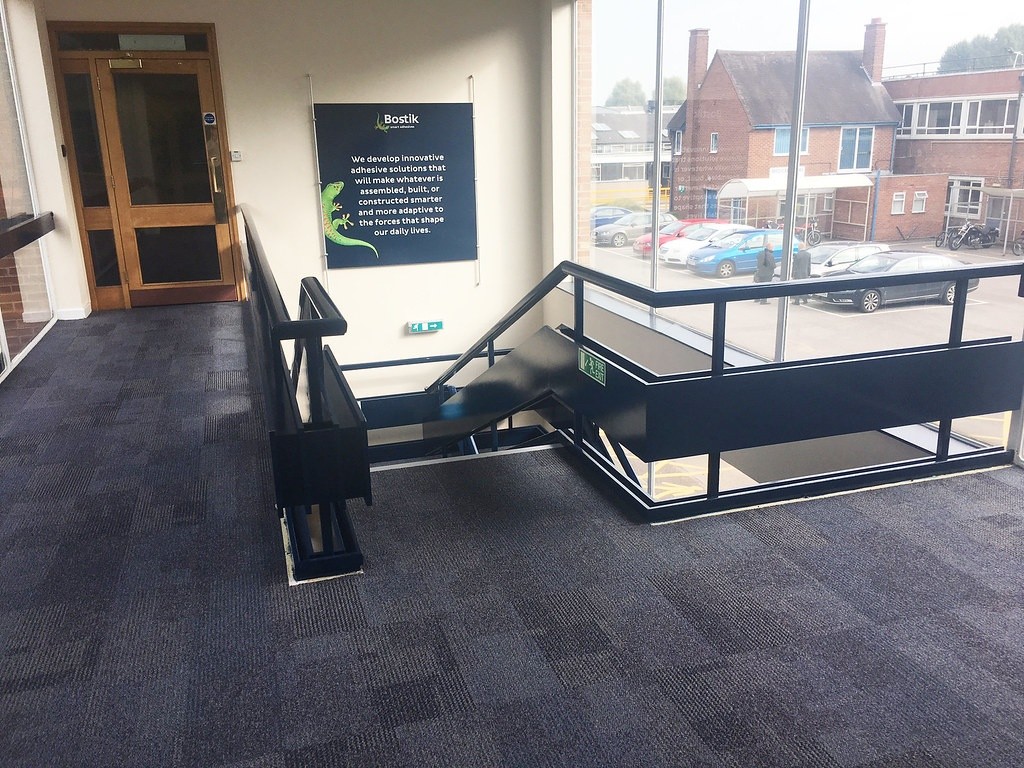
[760,301,770,305]
[792,299,800,305]
[754,300,761,302]
[803,299,807,303]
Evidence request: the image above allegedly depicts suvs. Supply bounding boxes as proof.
[633,217,732,256]
[589,211,679,247]
[772,241,890,286]
[657,222,756,268]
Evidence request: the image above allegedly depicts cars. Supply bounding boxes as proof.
[685,230,806,278]
[811,251,980,312]
[590,206,633,229]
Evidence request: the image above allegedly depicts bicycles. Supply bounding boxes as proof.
[1012,230,1024,256]
[936,226,962,248]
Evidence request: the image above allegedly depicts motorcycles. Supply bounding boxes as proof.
[762,215,821,246]
[948,217,1000,249]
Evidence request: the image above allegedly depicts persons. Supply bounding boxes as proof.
[753,242,777,304]
[791,242,811,305]
